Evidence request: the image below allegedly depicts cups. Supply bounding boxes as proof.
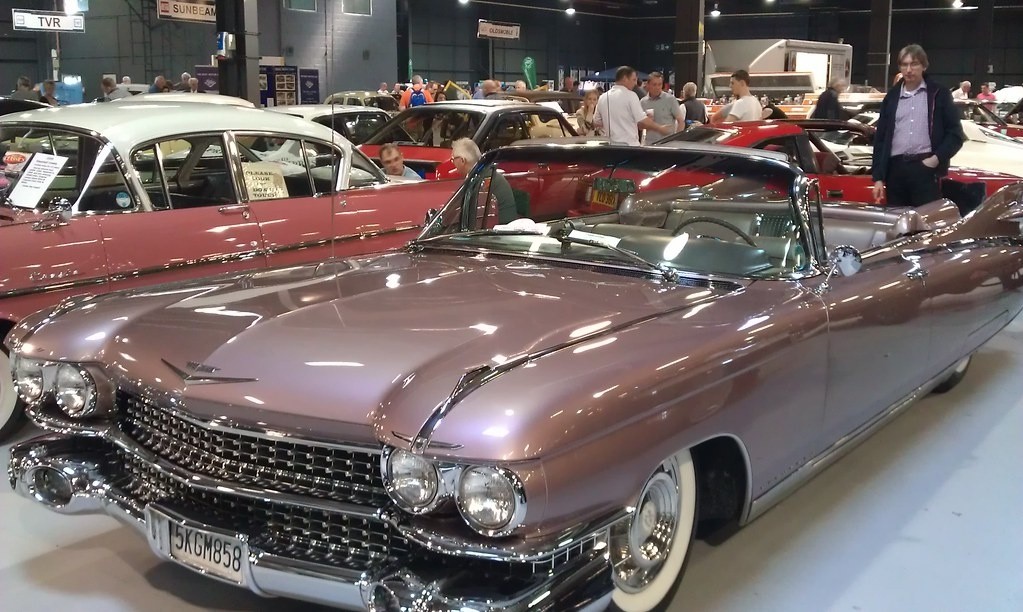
[646,109,654,120]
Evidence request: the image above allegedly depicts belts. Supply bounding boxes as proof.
[895,152,934,162]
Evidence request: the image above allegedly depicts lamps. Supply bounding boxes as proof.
[565,3,575,14]
[711,2,721,16]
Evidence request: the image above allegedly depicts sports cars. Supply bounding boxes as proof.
[0,141,1023,612]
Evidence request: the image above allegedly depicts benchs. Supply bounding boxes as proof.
[665,212,900,255]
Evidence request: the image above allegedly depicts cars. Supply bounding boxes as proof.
[0,84,1023,445]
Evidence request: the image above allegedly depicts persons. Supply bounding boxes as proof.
[976,83,996,112]
[377,63,996,161]
[640,71,685,145]
[8,72,205,105]
[675,82,708,134]
[379,142,423,180]
[951,81,971,100]
[449,137,518,224]
[872,42,967,207]
[591,65,674,146]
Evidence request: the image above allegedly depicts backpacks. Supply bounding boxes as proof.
[409,88,426,107]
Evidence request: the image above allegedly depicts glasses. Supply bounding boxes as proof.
[450,156,461,163]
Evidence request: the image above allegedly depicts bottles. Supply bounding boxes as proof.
[715,93,803,107]
